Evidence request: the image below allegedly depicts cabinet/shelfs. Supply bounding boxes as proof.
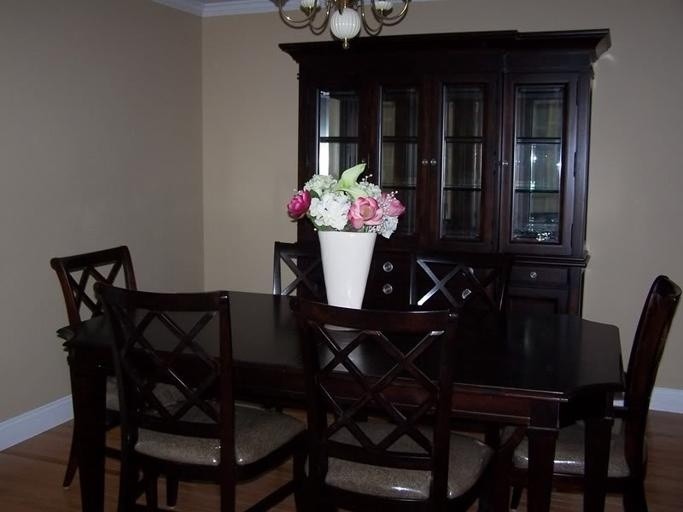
[281,34,429,247]
[295,255,589,318]
[429,28,614,260]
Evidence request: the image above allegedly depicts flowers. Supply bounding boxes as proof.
[282,161,406,240]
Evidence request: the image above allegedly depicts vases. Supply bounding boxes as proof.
[317,229,376,330]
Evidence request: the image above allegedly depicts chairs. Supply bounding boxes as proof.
[92,281,306,512]
[49,243,193,505]
[298,299,498,512]
[272,240,327,303]
[407,256,510,443]
[503,274,683,510]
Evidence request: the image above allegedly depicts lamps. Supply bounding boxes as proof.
[270,1,411,51]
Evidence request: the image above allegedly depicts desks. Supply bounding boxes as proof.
[55,288,626,512]
